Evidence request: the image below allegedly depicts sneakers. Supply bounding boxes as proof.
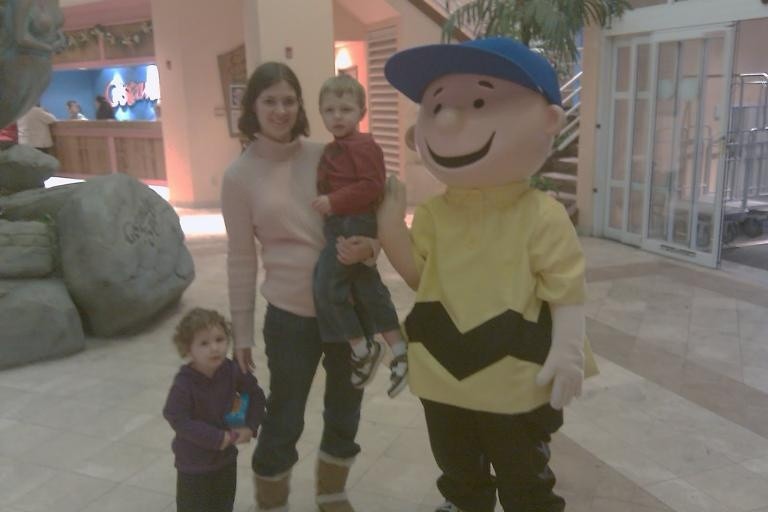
[346,341,382,390]
[384,351,411,401]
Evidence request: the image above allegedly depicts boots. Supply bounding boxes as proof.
[249,466,294,512]
[313,443,361,512]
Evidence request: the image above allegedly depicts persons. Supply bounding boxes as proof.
[309,75,412,399]
[376,37,603,512]
[92,95,115,120]
[65,99,88,118]
[162,308,267,512]
[16,102,58,157]
[220,63,384,511]
[0,118,19,145]
